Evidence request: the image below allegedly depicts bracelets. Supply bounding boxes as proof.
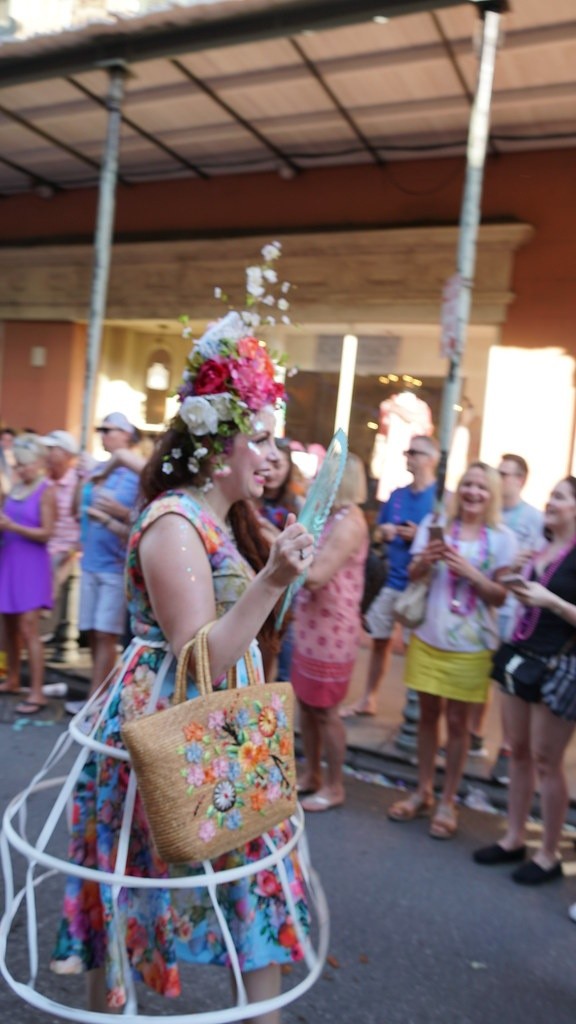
[101,517,112,527]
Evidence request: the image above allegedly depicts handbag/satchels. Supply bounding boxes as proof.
[120,620,299,864]
[489,642,550,701]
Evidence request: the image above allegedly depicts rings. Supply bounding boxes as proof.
[300,549,307,559]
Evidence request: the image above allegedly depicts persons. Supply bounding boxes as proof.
[387,461,515,839]
[338,434,453,717]
[439,453,544,788]
[250,438,371,813]
[53,405,317,1024]
[474,478,576,884]
[0,412,152,717]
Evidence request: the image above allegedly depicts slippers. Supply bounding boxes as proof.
[16,697,47,715]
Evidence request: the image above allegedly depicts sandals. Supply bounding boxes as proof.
[297,796,342,812]
[427,809,459,838]
[293,781,317,794]
[387,796,435,821]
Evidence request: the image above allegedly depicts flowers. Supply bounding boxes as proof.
[154,312,286,493]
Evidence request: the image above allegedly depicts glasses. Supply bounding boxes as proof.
[405,449,429,458]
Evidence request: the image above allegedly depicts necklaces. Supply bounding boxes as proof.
[512,537,576,640]
[447,520,489,617]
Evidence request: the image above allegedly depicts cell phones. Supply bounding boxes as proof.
[500,575,527,592]
[429,526,443,543]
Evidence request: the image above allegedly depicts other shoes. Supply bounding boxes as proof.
[471,843,526,864]
[511,855,564,886]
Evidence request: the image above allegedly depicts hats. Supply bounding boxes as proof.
[40,430,80,454]
[94,412,135,435]
